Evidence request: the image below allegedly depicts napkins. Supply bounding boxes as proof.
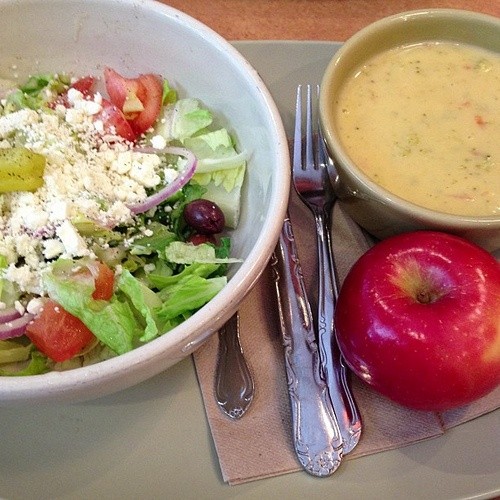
[191,132,500,487]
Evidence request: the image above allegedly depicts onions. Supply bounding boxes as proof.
[0,313,36,339]
[0,306,22,324]
[127,146,198,213]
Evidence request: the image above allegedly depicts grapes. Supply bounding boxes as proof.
[182,198,225,233]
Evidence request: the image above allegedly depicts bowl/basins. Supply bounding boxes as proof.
[1,0,292,407]
[318,10,500,248]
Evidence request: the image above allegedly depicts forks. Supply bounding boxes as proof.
[291,83,363,457]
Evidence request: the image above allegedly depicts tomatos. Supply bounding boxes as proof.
[49,76,95,110]
[94,97,136,146]
[24,264,115,361]
[186,232,216,244]
[105,68,163,133]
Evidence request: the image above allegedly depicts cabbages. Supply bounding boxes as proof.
[0,71,249,376]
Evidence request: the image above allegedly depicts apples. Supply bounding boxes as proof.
[334,231,500,410]
